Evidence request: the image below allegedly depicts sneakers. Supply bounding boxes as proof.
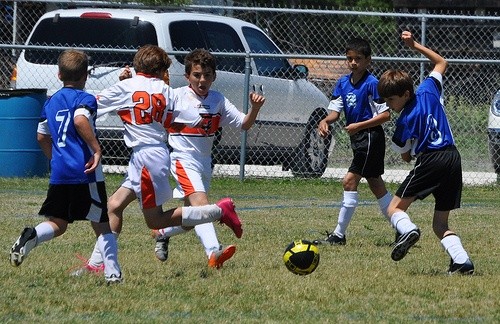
[312,231,346,246]
[216,197,243,238]
[9,228,38,267]
[447,257,474,275]
[389,224,420,261]
[105,272,124,286]
[207,244,236,270]
[152,229,170,261]
[68,254,105,278]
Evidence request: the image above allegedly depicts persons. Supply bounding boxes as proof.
[313,37,402,246]
[377,30,475,275]
[70,43,243,277]
[10,49,124,284]
[119,47,266,270]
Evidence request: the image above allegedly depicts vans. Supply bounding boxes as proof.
[6,5,337,178]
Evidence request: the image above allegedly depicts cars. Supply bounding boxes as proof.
[486,89,500,184]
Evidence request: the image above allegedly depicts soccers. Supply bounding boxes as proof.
[282,239,321,276]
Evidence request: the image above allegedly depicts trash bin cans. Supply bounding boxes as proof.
[0,88,50,178]
